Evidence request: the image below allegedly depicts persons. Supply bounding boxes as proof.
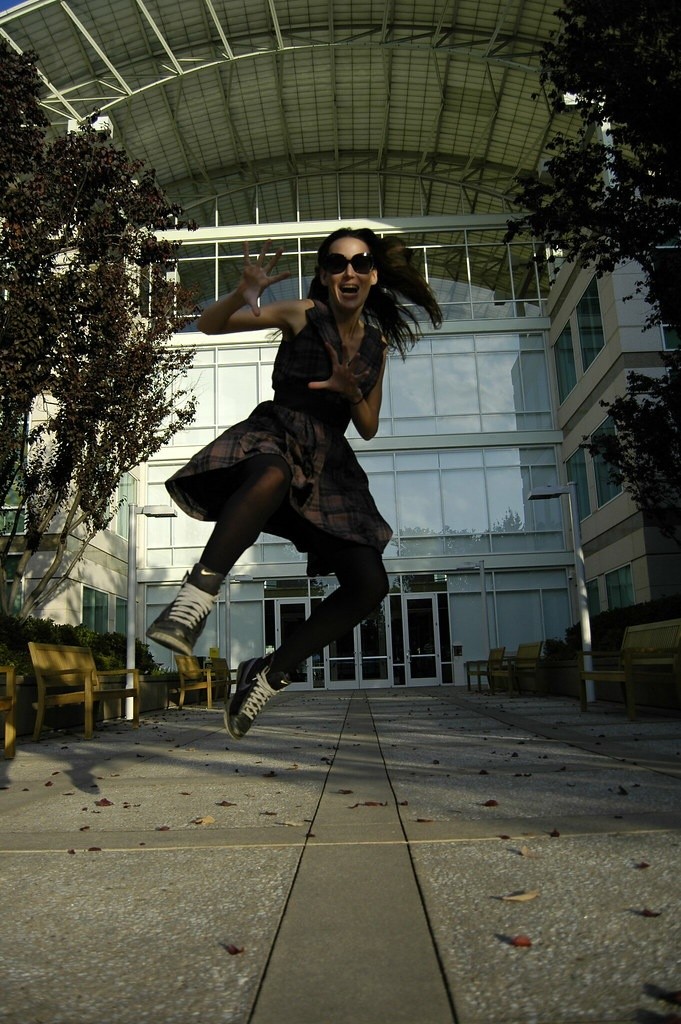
[145,226,449,736]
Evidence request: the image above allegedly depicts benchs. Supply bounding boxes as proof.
[211,657,237,701]
[27,641,153,739]
[577,618,681,722]
[0,664,24,761]
[486,641,546,695]
[174,652,231,708]
[463,646,505,694]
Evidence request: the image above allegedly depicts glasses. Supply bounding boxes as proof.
[318,253,375,274]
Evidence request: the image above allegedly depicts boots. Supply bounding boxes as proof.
[145,564,223,656]
[224,657,291,739]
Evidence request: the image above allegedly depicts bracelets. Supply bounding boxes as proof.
[349,396,364,408]
